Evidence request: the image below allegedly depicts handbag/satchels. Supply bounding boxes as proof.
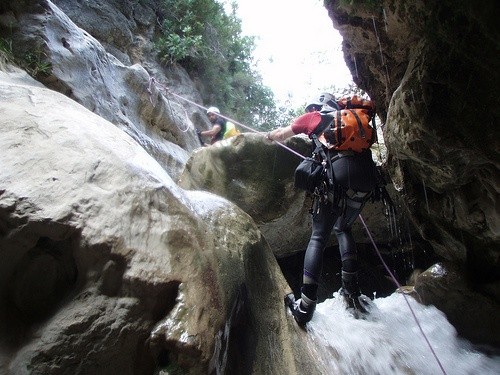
[294,157,323,194]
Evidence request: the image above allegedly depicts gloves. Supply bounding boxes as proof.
[199,136,205,144]
[196,128,201,135]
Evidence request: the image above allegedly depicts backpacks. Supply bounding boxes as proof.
[319,96,378,154]
[214,119,241,141]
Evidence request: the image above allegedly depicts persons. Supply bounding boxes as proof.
[200,106,226,145]
[263,92,381,326]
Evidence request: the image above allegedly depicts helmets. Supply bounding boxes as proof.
[305,92,341,112]
[205,106,221,116]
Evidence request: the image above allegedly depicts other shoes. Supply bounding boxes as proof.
[339,287,368,312]
[293,292,317,325]
